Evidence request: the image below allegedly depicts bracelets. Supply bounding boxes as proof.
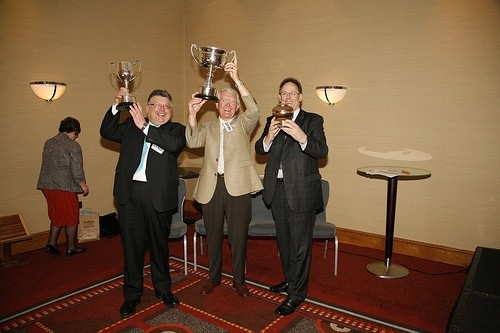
[234,80,244,87]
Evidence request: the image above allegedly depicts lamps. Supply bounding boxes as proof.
[29,81,67,102]
[316,84,347,105]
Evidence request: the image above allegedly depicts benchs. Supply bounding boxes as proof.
[194,179,338,277]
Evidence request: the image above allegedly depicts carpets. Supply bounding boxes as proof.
[0,255,425,333]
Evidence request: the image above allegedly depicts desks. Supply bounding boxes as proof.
[177,167,201,223]
[357,166,432,278]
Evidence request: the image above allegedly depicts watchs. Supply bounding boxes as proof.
[141,122,148,131]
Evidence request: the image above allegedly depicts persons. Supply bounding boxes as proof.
[37,116,89,255]
[185,55,264,298]
[101,88,187,317]
[254,77,328,315]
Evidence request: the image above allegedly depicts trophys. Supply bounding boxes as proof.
[272,102,294,128]
[109,59,141,112]
[190,43,236,101]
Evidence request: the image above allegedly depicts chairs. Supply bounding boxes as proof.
[165,178,188,276]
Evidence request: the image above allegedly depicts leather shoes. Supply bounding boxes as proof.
[270,281,289,293]
[66,247,87,256]
[275,297,300,316]
[120,296,140,318]
[200,281,218,295]
[233,282,250,297]
[45,244,62,256]
[155,290,179,307]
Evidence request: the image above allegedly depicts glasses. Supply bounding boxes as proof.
[282,91,301,96]
[147,102,171,109]
[220,99,237,104]
